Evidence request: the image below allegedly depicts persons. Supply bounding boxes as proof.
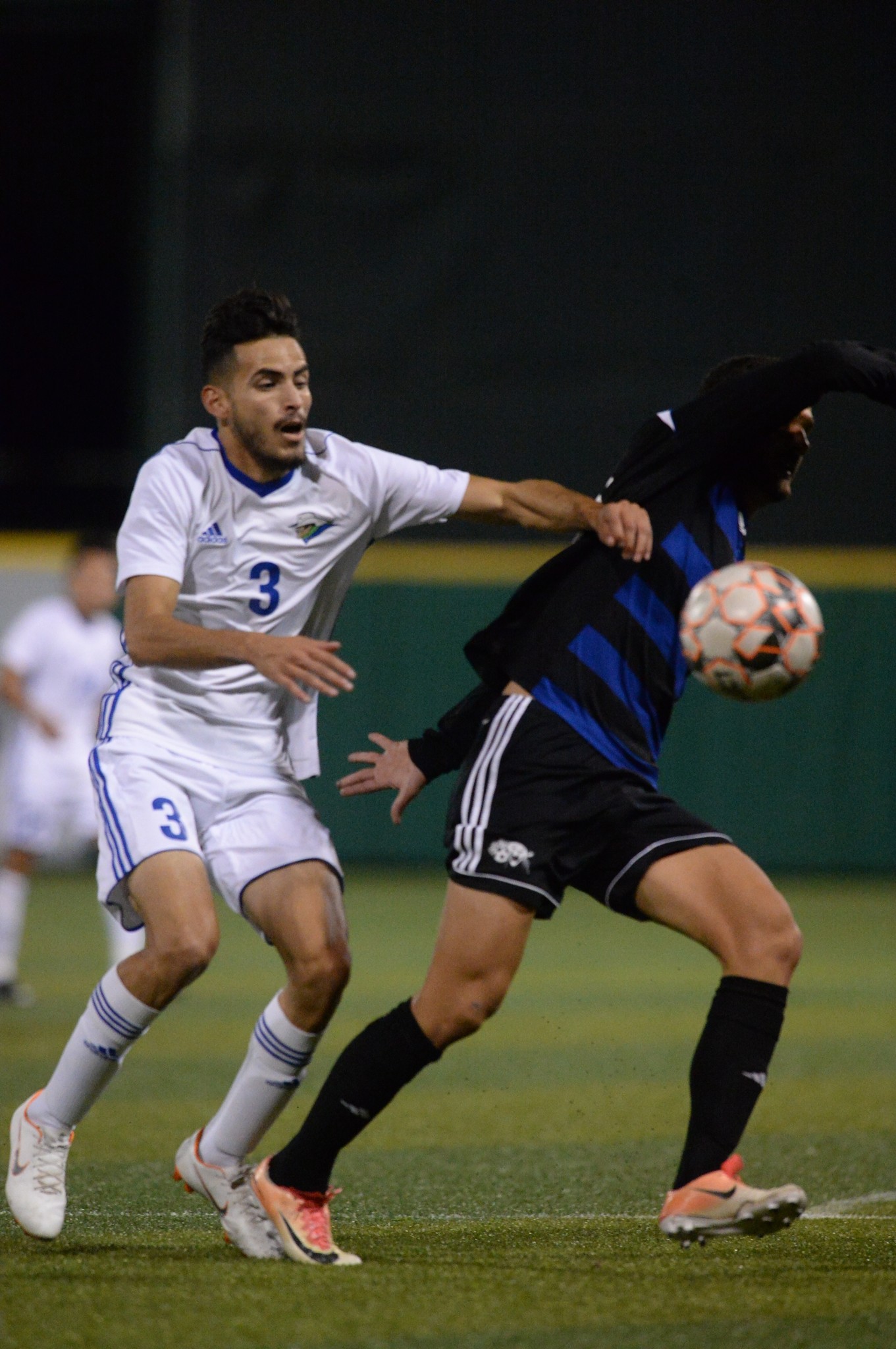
[3,286,652,1264]
[0,527,155,1037]
[246,334,895,1269]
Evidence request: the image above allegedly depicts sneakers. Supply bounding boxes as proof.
[175,1127,286,1263]
[6,1087,75,1239]
[659,1154,811,1250]
[248,1155,363,1266]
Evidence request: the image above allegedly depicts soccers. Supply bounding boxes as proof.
[680,562,824,700]
